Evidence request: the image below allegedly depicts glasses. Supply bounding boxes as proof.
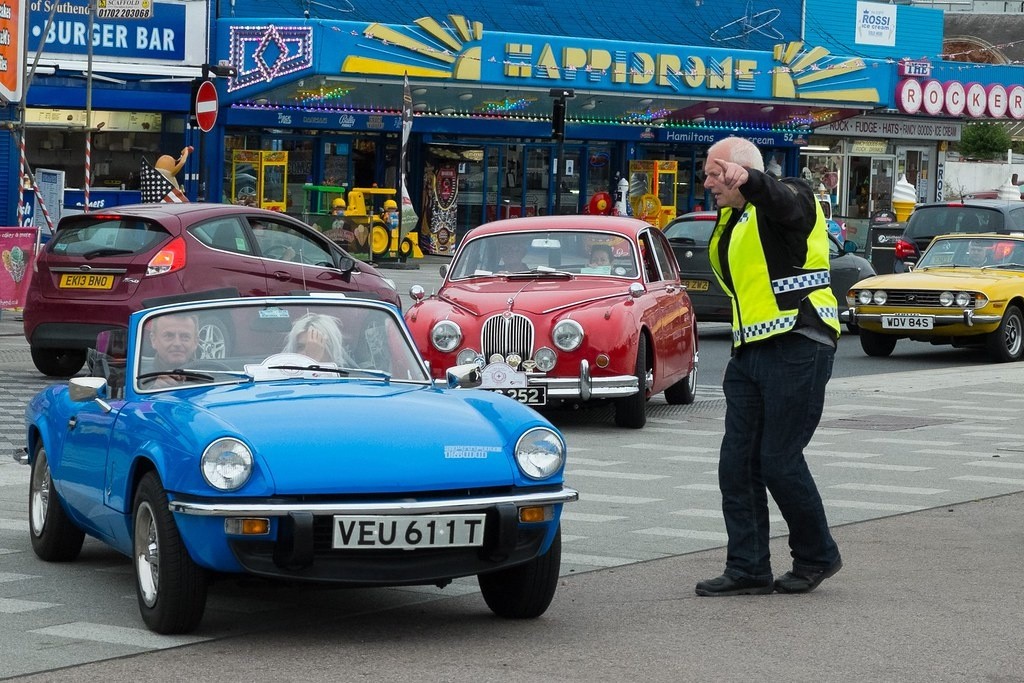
[590,257,609,264]
[502,245,524,253]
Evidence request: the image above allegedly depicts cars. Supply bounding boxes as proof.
[662,210,878,336]
[840,180,1024,362]
[403,214,699,429]
[22,201,402,377]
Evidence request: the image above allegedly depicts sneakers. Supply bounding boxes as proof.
[695,574,773,597]
[774,554,843,594]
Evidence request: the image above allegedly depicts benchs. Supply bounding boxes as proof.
[95,354,269,399]
[959,215,1001,231]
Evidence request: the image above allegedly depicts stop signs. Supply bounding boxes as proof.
[194,80,219,133]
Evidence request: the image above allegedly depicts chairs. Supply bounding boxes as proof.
[212,224,238,249]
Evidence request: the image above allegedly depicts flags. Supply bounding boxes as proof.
[137,156,190,203]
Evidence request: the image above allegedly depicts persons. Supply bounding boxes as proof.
[250,221,295,261]
[587,244,614,269]
[695,136,843,598]
[494,237,530,272]
[138,312,202,390]
[279,312,347,366]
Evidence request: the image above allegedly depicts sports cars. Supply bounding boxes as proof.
[25,292,579,636]
